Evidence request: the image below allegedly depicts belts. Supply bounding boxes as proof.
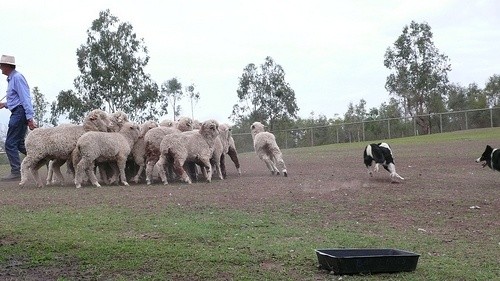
[11,105,23,112]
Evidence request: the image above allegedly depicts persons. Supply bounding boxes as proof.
[0,54,38,180]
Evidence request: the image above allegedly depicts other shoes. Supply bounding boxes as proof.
[2,174,19,181]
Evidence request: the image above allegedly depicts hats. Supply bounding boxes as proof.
[0,55,16,65]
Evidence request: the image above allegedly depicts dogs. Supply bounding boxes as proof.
[475,145,500,172]
[363,142,404,182]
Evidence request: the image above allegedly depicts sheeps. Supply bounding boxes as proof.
[156,119,220,186]
[250,122,288,177]
[219,123,241,180]
[19,109,113,188]
[73,121,142,188]
[110,104,204,186]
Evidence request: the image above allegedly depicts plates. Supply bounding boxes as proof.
[316,248,420,276]
